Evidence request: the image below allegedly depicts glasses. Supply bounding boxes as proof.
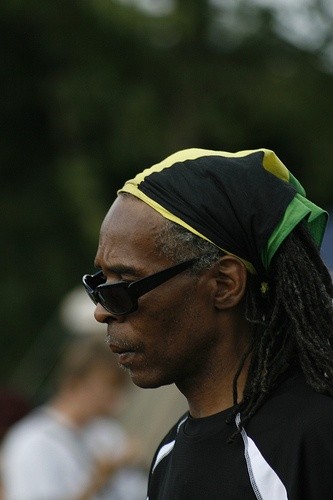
[82,258,196,316]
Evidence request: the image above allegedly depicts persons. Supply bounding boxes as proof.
[0,286,153,500]
[83,149,332,500]
